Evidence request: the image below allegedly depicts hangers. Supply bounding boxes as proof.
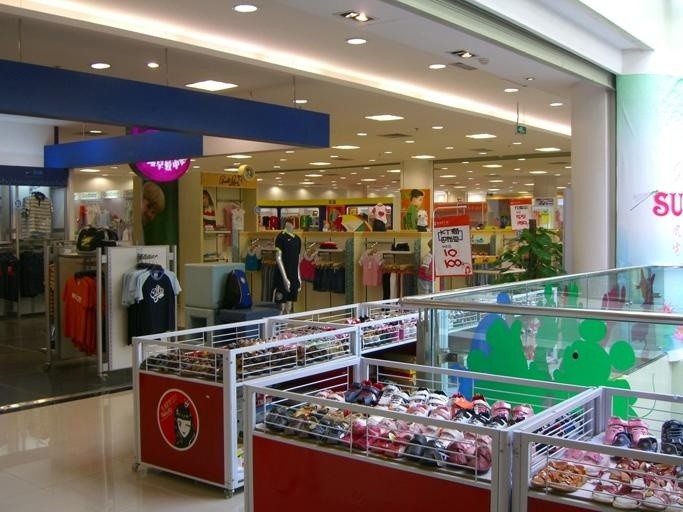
[135,252,168,274]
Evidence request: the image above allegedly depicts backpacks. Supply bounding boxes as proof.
[75,226,120,253]
[222,269,253,310]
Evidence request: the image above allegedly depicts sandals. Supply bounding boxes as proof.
[136,305,423,384]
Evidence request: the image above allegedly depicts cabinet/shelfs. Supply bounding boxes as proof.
[178,172,259,313]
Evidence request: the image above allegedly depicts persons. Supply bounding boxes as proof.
[415,208,428,232]
[141,181,165,224]
[270,220,302,315]
[417,239,437,297]
[371,202,387,232]
[405,188,424,231]
[203,188,218,230]
